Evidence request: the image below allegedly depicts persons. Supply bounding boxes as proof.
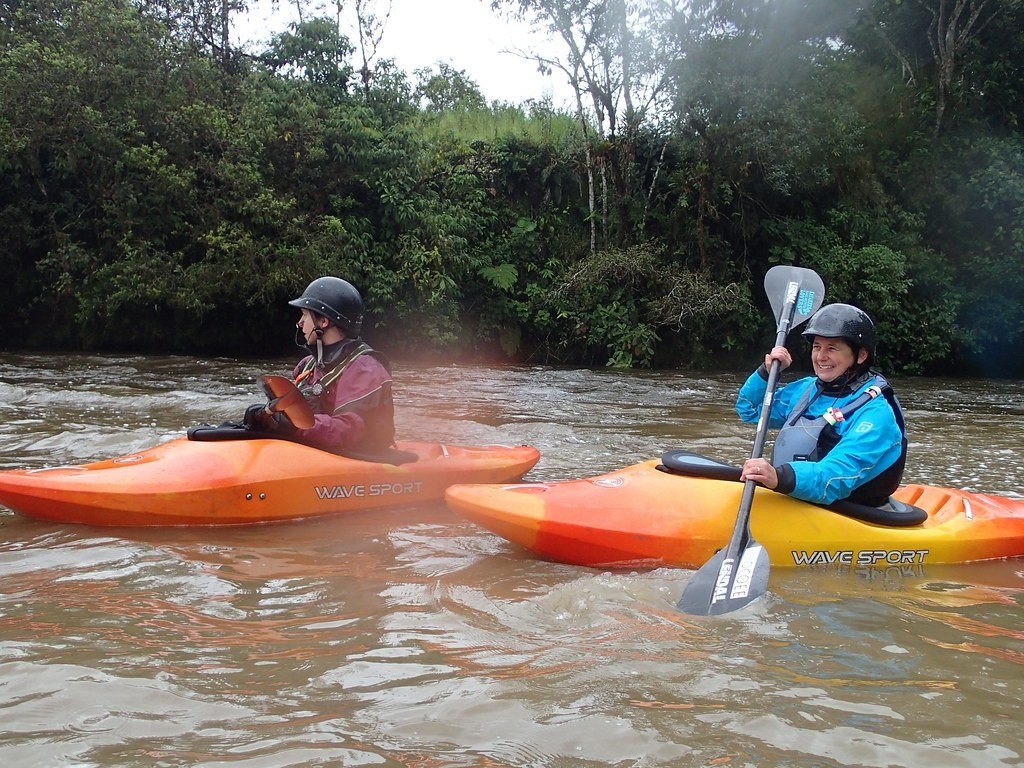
[737,302,906,517]
[241,277,394,460]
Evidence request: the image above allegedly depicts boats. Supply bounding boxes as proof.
[443,449,1024,569]
[0,435,540,527]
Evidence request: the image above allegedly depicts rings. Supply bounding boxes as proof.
[757,466,760,475]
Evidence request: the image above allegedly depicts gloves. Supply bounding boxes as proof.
[244,404,282,431]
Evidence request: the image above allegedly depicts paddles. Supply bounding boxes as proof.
[198,373,317,430]
[673,263,828,617]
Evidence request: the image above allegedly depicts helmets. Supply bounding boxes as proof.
[288,276,363,334]
[802,303,877,366]
